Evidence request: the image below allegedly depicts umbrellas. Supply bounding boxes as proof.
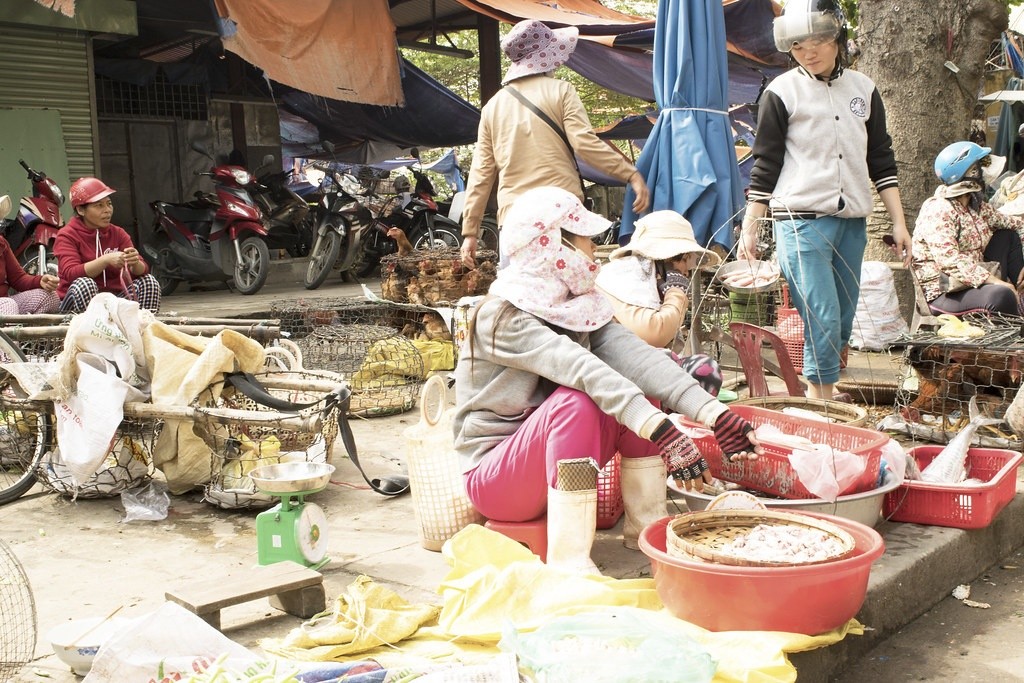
[618,0,746,355]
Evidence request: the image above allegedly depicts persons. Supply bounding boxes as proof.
[52,178,162,314]
[912,141,1024,324]
[0,195,60,316]
[595,209,724,398]
[460,19,651,271]
[453,187,765,581]
[737,0,913,400]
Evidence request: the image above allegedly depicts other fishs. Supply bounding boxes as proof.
[917,393,1006,485]
[720,269,779,288]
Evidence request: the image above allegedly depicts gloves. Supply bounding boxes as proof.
[658,273,691,294]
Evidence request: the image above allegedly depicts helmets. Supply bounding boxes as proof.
[934,142,993,184]
[69,177,116,208]
[781,0,844,42]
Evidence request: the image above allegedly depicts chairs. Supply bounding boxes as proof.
[728,321,854,403]
[907,262,946,357]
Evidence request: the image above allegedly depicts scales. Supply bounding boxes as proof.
[252,460,335,569]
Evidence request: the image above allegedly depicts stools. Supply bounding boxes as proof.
[166,559,327,630]
[484,514,548,564]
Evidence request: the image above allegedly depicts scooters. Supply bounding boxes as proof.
[140,141,276,297]
[0,159,65,297]
[191,141,772,291]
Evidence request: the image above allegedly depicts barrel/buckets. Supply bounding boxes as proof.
[729,290,767,327]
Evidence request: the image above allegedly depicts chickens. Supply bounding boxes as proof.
[379,226,499,340]
[900,347,1024,428]
[296,297,345,333]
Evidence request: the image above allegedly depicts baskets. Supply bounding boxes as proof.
[679,402,1022,528]
[777,283,849,373]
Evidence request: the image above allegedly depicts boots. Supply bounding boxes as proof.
[616,454,671,549]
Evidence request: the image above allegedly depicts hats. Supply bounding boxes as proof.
[499,19,580,85]
[607,210,722,271]
[0,194,12,219]
[503,186,612,259]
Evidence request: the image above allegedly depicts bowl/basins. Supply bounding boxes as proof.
[716,259,780,293]
[663,471,900,528]
[50,617,120,676]
[637,508,886,637]
